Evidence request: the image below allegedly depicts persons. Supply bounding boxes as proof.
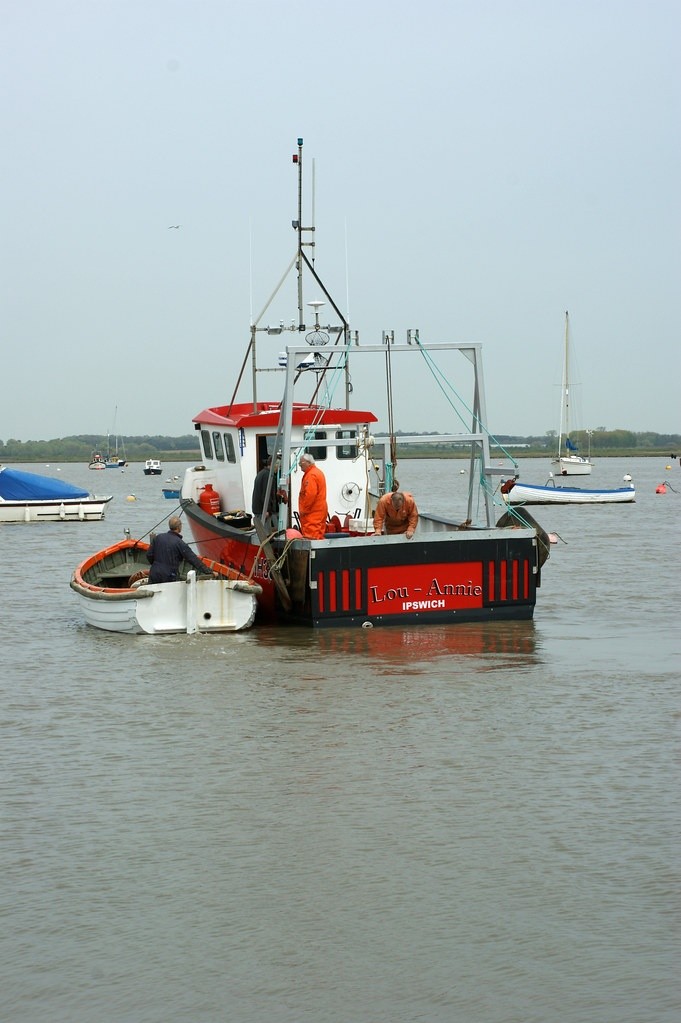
[371,492,418,539]
[146,516,219,584]
[252,455,284,530]
[298,454,327,541]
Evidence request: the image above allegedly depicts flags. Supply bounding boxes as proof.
[566,438,577,450]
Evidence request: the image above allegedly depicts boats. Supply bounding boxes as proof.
[143,457,163,476]
[500,472,638,507]
[0,467,114,523]
[161,488,185,500]
[65,526,262,638]
[178,137,561,629]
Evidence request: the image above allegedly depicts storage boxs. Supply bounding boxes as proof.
[217,513,253,527]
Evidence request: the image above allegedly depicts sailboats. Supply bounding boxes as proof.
[86,404,129,469]
[551,310,600,475]
